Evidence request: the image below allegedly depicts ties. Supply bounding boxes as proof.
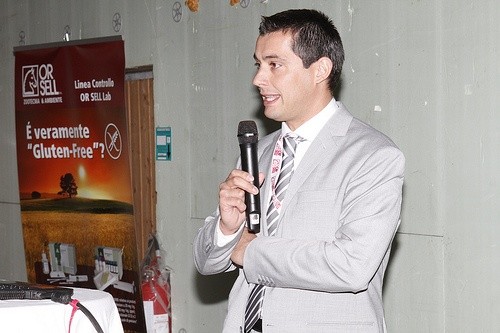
[243,134,306,332]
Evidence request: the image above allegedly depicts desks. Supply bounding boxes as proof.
[0,286,125,333]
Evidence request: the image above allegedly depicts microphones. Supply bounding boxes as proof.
[237,120,261,234]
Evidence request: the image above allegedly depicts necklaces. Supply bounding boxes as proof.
[270,132,289,213]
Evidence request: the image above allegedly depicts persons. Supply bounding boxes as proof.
[193,9,406,333]
